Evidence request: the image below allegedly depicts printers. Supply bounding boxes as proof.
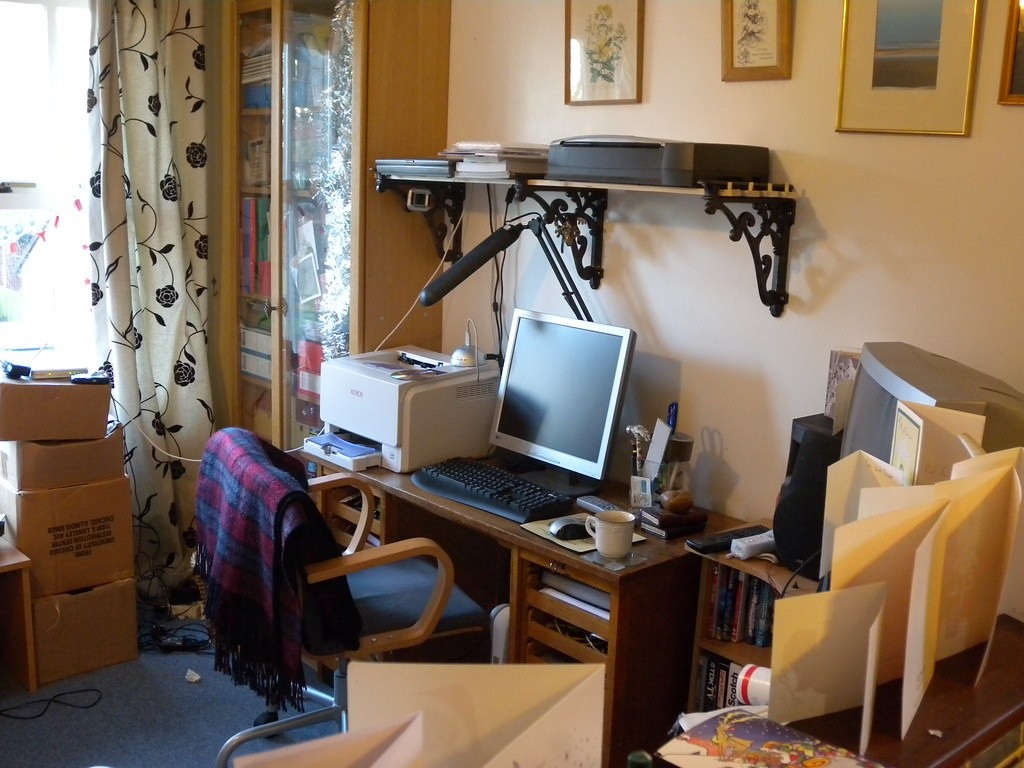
[303,344,499,474]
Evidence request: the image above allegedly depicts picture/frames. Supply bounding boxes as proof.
[833,0,985,139]
[720,0,793,84]
[994,0,1024,107]
[562,0,644,107]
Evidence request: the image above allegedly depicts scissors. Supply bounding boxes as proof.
[667,401,679,436]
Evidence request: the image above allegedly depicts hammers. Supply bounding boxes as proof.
[626,424,651,477]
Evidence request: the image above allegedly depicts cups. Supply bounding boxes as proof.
[586,512,635,558]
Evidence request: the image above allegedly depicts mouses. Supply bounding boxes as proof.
[549,517,596,540]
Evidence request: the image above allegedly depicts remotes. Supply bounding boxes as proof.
[686,525,772,555]
[576,496,627,515]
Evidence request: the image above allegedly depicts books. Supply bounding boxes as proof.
[706,557,774,648]
[652,709,882,768]
[439,139,545,181]
[693,654,743,711]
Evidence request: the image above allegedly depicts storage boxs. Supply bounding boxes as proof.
[0,366,121,444]
[0,415,129,491]
[33,575,140,686]
[0,470,136,598]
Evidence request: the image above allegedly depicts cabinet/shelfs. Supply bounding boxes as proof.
[684,518,820,715]
[223,0,451,450]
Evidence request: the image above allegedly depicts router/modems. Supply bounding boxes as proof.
[31,343,88,379]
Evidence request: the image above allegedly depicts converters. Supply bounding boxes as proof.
[159,636,186,650]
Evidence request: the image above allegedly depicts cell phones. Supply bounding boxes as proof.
[71,373,109,384]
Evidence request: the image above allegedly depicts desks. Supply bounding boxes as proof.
[286,444,748,768]
[0,535,39,690]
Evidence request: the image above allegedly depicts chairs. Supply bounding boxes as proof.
[189,424,490,768]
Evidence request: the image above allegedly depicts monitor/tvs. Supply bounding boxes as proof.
[488,308,637,498]
[839,342,1024,460]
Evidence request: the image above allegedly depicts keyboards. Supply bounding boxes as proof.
[410,456,574,524]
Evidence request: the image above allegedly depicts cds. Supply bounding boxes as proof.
[391,370,433,380]
